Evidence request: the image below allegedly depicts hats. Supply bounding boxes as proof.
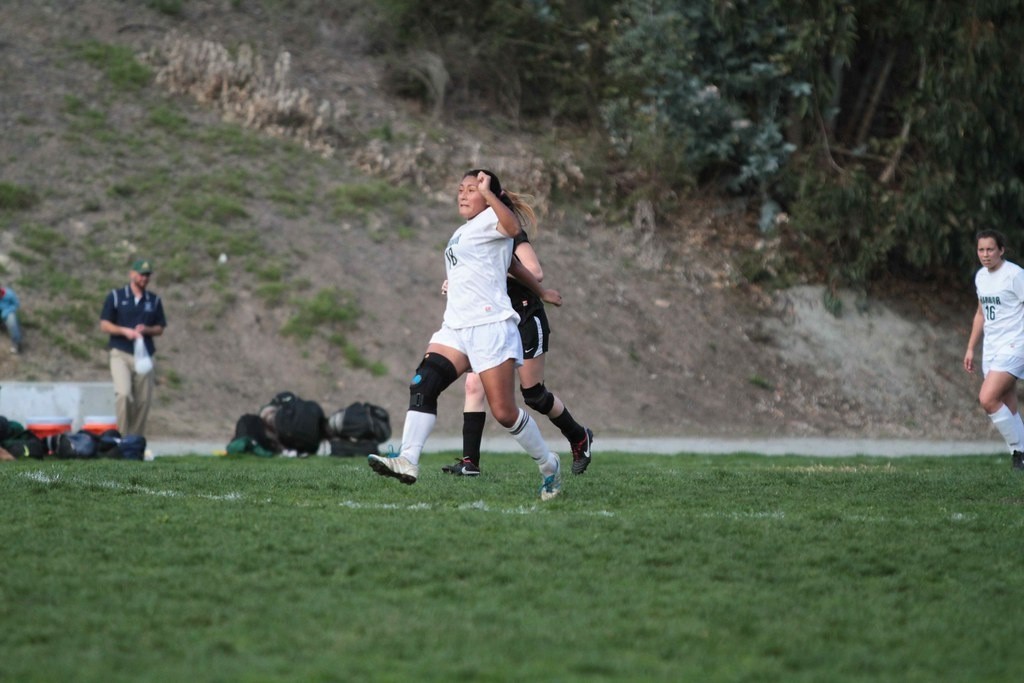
[131,259,152,275]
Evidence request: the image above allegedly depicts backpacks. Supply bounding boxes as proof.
[0,416,146,461]
[226,392,391,457]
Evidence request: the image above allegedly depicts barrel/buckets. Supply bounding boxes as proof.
[21,416,74,454]
[84,413,121,434]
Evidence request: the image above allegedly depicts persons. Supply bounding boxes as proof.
[442,191,593,476]
[100,259,167,437]
[369,169,563,502]
[963,230,1024,470]
[0,286,22,353]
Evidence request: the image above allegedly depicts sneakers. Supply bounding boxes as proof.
[539,453,561,502]
[442,455,480,478]
[368,454,420,485]
[571,426,593,476]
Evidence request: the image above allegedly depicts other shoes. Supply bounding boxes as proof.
[1012,449,1024,471]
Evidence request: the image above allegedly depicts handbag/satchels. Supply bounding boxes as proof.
[133,334,152,376]
